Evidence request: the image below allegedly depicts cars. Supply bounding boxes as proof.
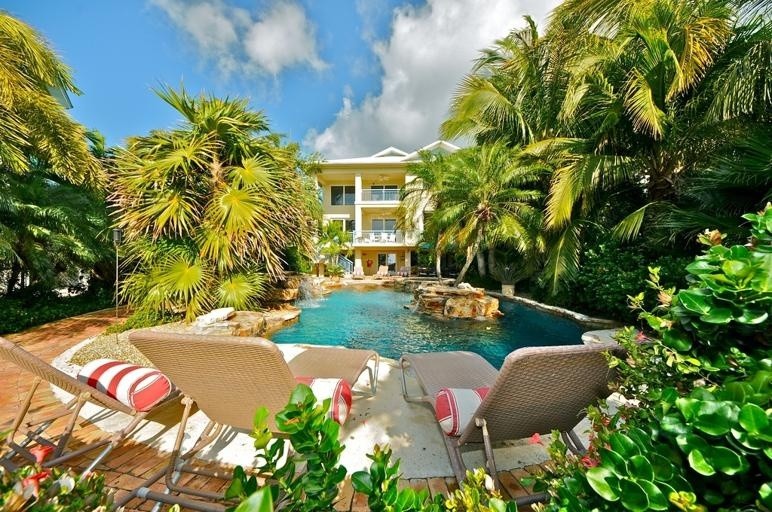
[416,267,429,277]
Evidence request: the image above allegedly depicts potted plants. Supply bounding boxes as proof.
[325,263,344,282]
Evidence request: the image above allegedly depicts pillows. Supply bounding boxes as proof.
[293,377,353,426]
[435,387,492,437]
[77,358,172,411]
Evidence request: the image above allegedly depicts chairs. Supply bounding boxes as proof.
[0,337,183,489]
[107,331,379,512]
[398,266,409,277]
[399,344,610,512]
[376,264,388,276]
[369,232,396,242]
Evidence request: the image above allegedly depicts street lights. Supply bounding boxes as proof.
[111,227,121,318]
